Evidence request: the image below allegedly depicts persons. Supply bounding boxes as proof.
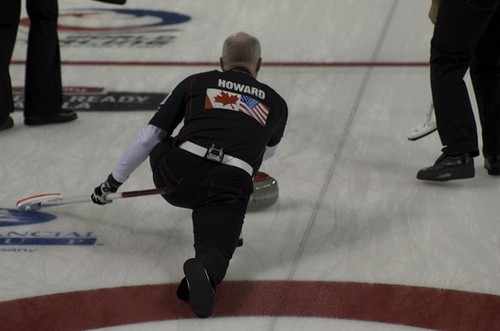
[0,0,78,132]
[91,32,288,318]
[414,0,500,182]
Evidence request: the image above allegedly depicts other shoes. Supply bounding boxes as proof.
[182,258,215,318]
[0,115,13,129]
[24,109,79,125]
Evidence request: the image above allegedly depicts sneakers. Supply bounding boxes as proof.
[484,155,500,176]
[416,152,474,180]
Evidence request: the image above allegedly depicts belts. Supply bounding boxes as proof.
[180,140,253,176]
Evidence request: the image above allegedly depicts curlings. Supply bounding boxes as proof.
[246,171,280,212]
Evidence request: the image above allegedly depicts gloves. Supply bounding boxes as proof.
[91,174,122,205]
